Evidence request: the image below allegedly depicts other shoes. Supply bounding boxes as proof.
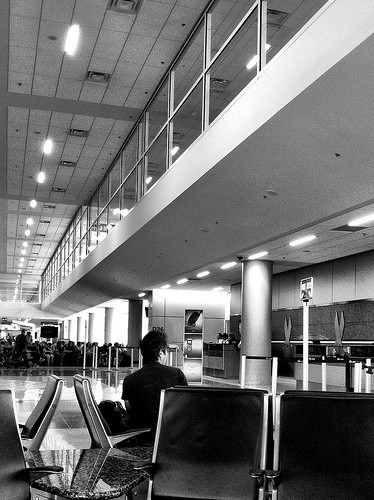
[115,401,126,417]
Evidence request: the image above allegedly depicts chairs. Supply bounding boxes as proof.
[73,375,151,448]
[133,385,374,500]
[0,374,64,500]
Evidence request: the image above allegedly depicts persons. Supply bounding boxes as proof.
[228,334,236,341]
[0,327,132,372]
[216,333,223,341]
[222,333,228,340]
[120,330,188,447]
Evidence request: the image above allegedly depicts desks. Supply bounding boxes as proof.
[25,448,153,500]
